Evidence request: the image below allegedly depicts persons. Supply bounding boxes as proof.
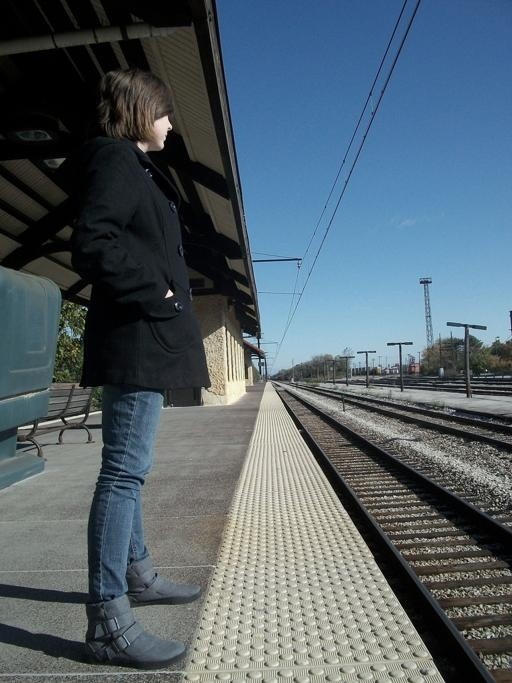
[71,63,203,668]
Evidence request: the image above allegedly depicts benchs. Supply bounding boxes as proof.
[16,386,99,462]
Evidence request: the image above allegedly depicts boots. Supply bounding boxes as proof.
[82,592,187,664]
[124,553,204,608]
